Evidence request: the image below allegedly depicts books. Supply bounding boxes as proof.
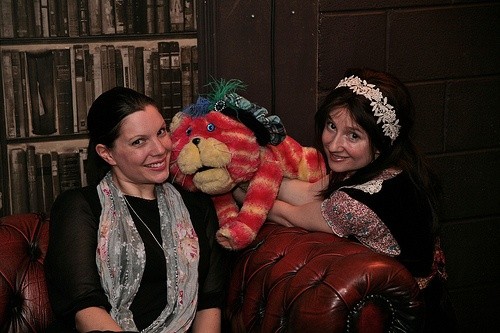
[0,41,197,139]
[0,0,198,39]
[9,145,89,214]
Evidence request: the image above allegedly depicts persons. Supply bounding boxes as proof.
[232,67,455,333]
[49,86,225,333]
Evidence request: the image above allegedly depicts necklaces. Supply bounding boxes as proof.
[123,188,165,252]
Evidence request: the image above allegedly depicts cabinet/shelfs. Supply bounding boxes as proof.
[0,0,205,212]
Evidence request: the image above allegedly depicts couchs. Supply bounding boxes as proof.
[0,206,428,333]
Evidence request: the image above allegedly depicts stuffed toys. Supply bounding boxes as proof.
[166,92,329,249]
